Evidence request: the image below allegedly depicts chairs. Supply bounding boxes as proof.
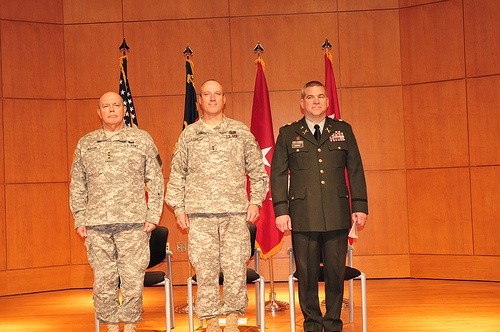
[287,244,369,332]
[94,225,176,332]
[186,224,266,332]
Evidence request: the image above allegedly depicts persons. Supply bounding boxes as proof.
[69,92,165,332]
[165,79,270,332]
[269,81,368,332]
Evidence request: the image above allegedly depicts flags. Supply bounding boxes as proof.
[181,58,198,131]
[118,54,149,205]
[324,49,355,249]
[245,60,290,260]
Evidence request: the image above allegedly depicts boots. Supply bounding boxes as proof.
[223,312,240,332]
[206,317,222,332]
[107,323,120,332]
[123,323,136,332]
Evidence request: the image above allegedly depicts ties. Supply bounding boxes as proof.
[314,125,322,143]
